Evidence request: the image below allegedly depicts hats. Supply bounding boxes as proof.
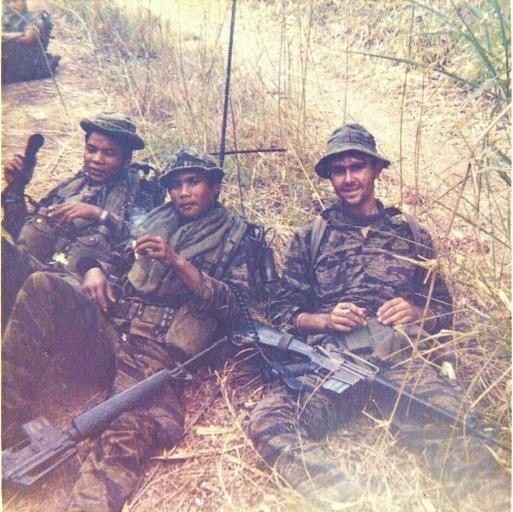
[158,146,225,186]
[80,110,145,150]
[314,123,391,179]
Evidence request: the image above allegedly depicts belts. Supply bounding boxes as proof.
[107,293,178,329]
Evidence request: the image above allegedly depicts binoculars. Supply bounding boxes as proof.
[251,247,277,285]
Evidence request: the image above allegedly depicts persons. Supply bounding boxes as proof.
[1,1,52,85]
[0,107,167,335]
[0,149,261,512]
[240,121,498,509]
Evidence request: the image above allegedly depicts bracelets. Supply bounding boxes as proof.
[98,208,109,223]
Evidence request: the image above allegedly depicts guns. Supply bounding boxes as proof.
[237,317,512,452]
[1,335,229,487]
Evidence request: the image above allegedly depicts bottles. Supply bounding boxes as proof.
[16,206,57,264]
